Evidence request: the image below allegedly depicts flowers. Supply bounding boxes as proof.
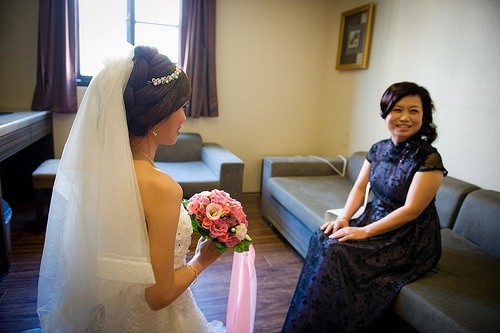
[181,188,257,253]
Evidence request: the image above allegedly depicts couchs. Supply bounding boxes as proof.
[261,150,500,333]
[31,132,245,201]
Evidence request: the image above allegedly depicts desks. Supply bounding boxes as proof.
[1,110,54,162]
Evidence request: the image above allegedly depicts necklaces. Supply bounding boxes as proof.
[131,146,157,167]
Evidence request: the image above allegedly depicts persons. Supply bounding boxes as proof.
[280,81,448,333]
[36,45,227,333]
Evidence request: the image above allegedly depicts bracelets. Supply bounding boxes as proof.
[187,264,198,278]
[336,217,350,220]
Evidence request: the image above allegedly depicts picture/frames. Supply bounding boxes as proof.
[335,4,376,70]
